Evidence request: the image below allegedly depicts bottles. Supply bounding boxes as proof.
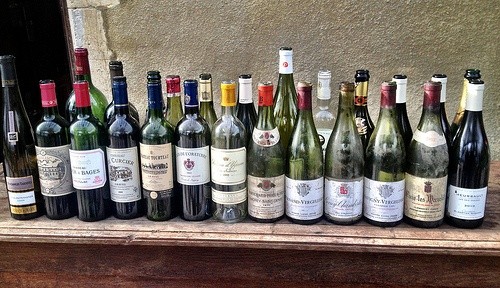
[285,79,324,225]
[0,55,45,221]
[247,81,285,223]
[68,80,111,223]
[105,76,144,220]
[138,80,177,221]
[324,81,365,226]
[174,78,213,221]
[63,47,108,130]
[363,82,406,227]
[404,81,450,229]
[311,69,336,156]
[391,73,414,155]
[103,59,139,132]
[273,46,299,151]
[431,68,490,229]
[209,79,248,223]
[353,69,375,149]
[145,69,258,143]
[35,78,75,220]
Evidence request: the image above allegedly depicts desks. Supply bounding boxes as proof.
[0,161,500,288]
